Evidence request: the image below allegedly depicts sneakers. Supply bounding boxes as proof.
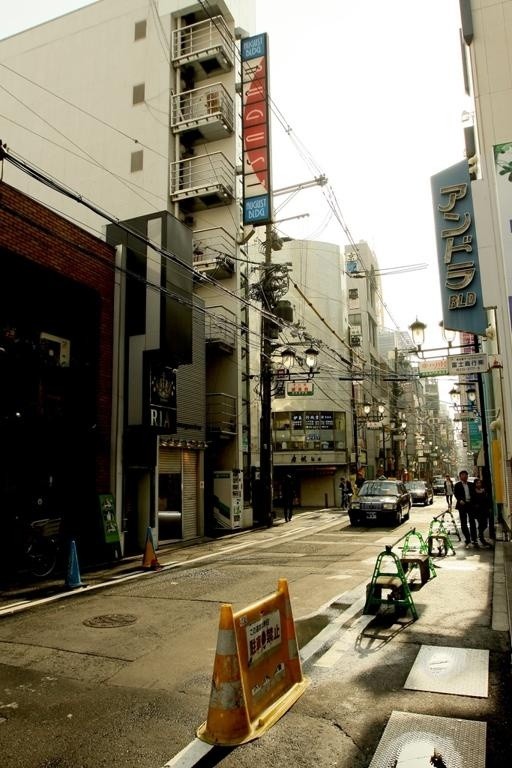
[489,532,495,538]
[471,537,478,542]
[479,532,483,538]
[465,539,470,543]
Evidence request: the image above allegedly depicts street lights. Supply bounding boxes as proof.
[258,334,322,528]
[405,309,498,541]
[410,432,460,477]
[450,378,481,422]
[351,397,386,487]
[379,415,408,480]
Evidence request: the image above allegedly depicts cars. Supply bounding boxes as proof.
[432,475,481,496]
[404,479,435,507]
[346,474,415,527]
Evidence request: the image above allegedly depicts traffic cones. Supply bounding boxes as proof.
[65,540,88,590]
[141,525,164,572]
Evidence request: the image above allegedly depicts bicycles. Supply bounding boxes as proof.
[1,514,61,578]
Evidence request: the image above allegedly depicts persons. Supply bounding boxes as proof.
[453,470,479,545]
[470,478,490,546]
[279,476,295,524]
[443,474,454,509]
[338,477,348,507]
[374,467,385,480]
[354,473,366,489]
[343,481,354,509]
